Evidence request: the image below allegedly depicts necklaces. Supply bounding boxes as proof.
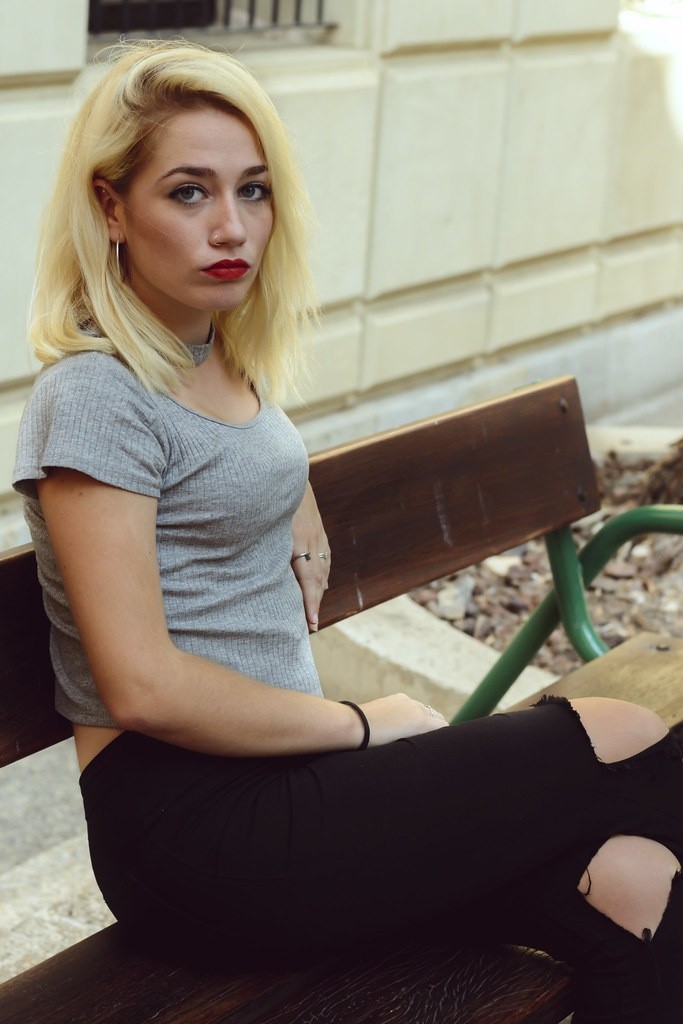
[188,322,217,368]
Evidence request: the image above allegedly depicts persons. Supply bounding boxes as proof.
[10,38,680,1024]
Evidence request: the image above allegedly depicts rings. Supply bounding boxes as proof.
[317,551,329,560]
[291,552,311,563]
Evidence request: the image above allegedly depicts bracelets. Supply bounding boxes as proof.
[337,700,371,751]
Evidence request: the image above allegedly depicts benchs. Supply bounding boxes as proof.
[1,373,683,1024]
